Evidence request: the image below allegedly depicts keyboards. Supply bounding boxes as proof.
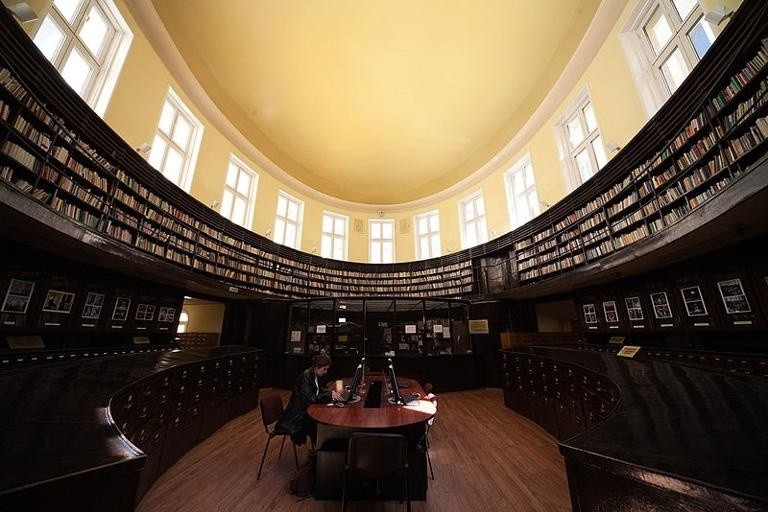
[402,394,420,406]
[333,390,352,404]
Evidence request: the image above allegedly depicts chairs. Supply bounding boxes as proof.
[423,382,433,395]
[340,430,412,511]
[423,392,440,481]
[256,391,314,481]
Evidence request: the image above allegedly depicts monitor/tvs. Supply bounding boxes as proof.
[386,358,408,404]
[347,357,366,403]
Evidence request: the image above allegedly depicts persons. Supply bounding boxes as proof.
[274,353,344,446]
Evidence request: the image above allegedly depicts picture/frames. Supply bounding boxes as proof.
[581,276,753,324]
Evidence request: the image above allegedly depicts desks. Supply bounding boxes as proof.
[305,370,438,430]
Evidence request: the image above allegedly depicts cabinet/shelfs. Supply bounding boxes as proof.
[692,37,767,181]
[497,346,768,511]
[0,3,257,286]
[257,232,511,305]
[614,126,728,246]
[511,196,628,284]
[0,346,262,512]
